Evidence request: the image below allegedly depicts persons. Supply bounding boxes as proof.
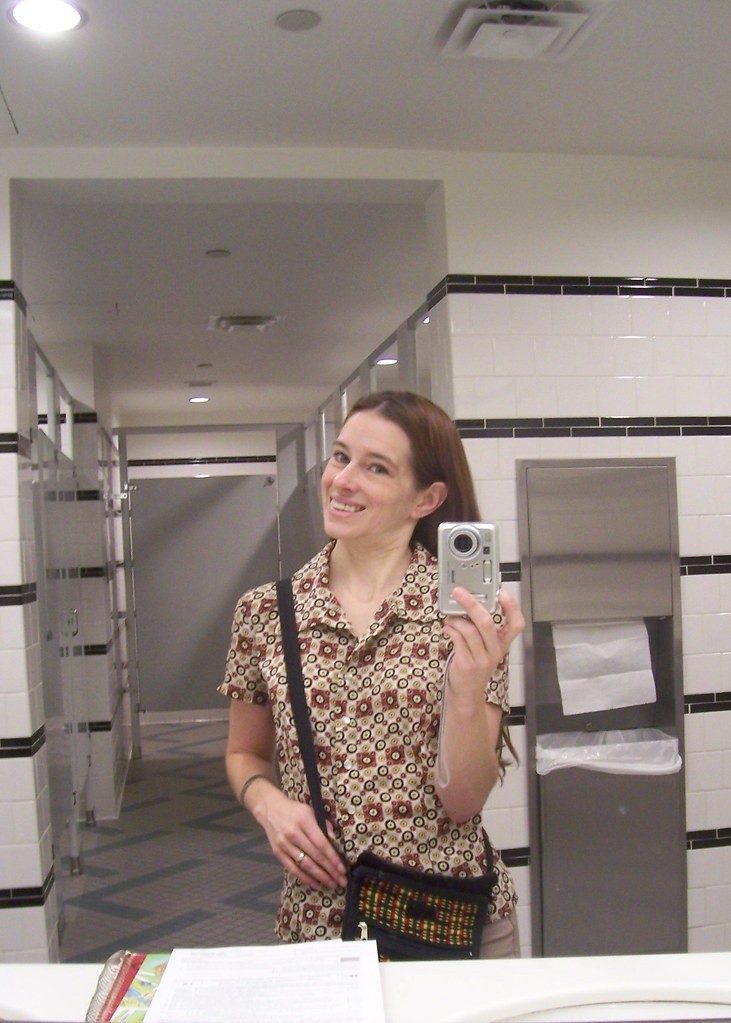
[215,392,527,961]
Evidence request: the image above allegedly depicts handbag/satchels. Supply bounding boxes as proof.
[342,851,497,959]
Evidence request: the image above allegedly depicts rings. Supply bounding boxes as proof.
[296,852,305,866]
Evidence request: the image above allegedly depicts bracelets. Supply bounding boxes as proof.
[240,775,276,809]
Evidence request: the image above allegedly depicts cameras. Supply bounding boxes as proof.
[436,522,503,616]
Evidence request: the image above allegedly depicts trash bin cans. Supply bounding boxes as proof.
[536,727,689,956]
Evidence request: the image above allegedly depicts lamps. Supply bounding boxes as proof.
[425,0,616,66]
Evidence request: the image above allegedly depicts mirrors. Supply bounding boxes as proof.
[1,0,731,964]
